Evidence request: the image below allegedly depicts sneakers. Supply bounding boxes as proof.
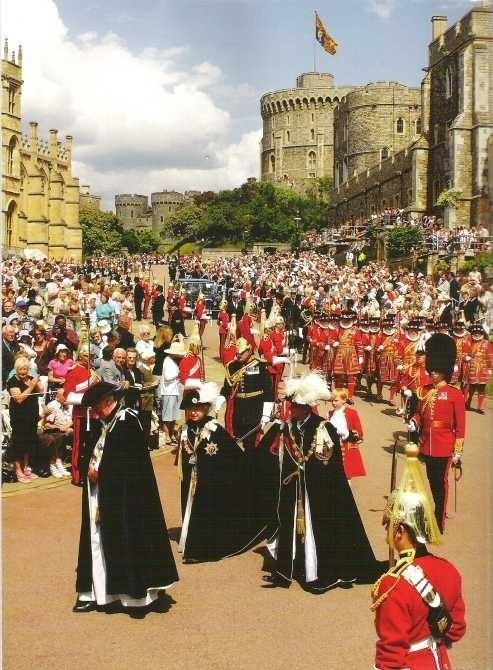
[2,462,71,483]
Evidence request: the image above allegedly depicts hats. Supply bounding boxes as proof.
[164,342,184,355]
[426,333,456,382]
[82,383,118,408]
[56,344,71,354]
[16,301,29,307]
[17,330,32,341]
[180,378,218,409]
[97,320,112,334]
[313,311,485,335]
[284,367,333,408]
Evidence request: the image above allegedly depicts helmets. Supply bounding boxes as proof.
[184,320,201,355]
[219,291,285,354]
[75,321,96,360]
[383,442,444,547]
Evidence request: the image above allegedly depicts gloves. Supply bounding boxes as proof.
[261,416,270,430]
[404,390,413,398]
[452,454,461,462]
[407,421,416,432]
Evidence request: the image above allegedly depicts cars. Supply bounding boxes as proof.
[168,277,225,321]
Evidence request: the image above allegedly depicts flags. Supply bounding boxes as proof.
[316,12,338,55]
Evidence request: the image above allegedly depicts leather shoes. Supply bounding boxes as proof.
[263,576,291,588]
[74,602,97,611]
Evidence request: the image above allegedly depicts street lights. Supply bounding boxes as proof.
[241,231,248,241]
[290,208,302,227]
